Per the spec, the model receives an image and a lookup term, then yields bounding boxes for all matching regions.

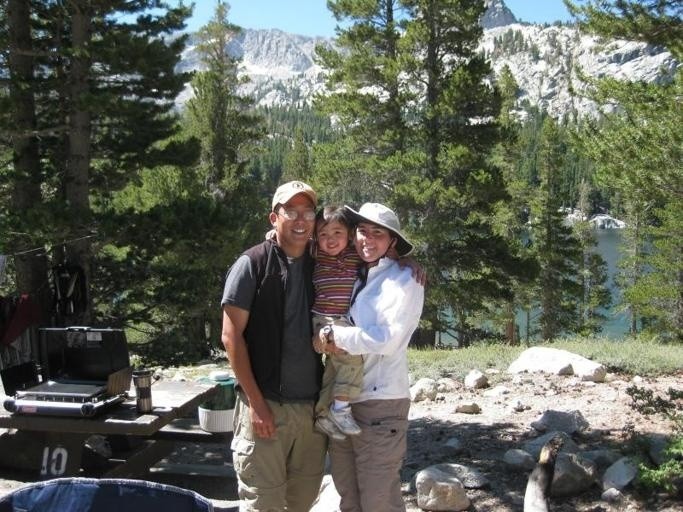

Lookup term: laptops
[13,326,128,403]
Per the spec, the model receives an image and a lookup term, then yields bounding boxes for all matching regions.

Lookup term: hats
[271,181,318,210]
[344,202,415,257]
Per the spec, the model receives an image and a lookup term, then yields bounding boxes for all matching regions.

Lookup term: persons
[219,179,428,512]
[263,203,399,443]
[312,202,424,512]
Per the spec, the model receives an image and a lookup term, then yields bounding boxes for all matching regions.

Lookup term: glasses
[274,210,317,221]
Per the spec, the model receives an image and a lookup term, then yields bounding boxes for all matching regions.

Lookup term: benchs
[0,418,232,446]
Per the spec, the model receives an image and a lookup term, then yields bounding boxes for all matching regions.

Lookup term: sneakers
[314,403,363,440]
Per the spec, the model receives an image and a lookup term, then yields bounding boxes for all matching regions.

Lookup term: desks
[0,380,221,480]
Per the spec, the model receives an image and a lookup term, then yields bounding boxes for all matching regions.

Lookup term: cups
[131,370,157,413]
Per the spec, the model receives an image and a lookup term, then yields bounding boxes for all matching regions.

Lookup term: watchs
[321,323,333,343]
